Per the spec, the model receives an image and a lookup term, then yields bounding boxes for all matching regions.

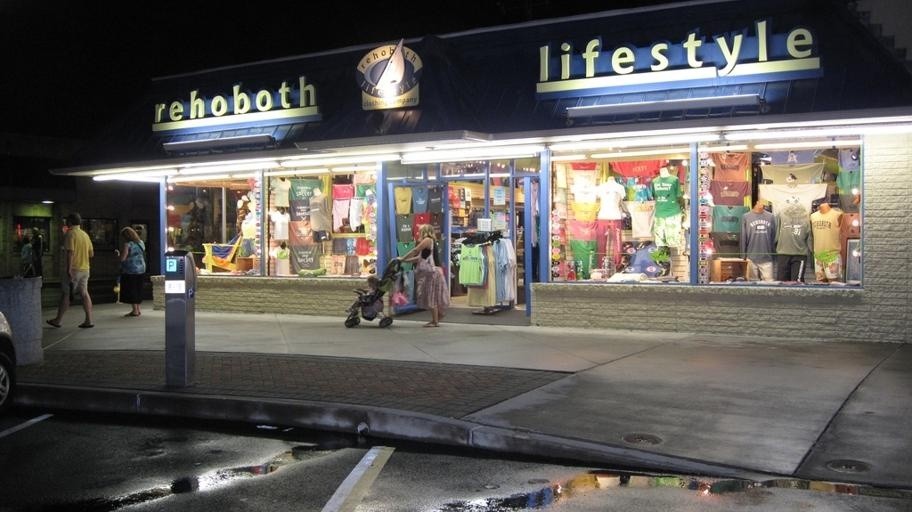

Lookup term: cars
[0,309,19,411]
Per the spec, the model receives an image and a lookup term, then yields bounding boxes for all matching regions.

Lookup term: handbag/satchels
[417,237,436,273]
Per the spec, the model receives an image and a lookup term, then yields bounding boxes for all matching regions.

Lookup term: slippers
[77,320,95,329]
[45,319,62,328]
[123,311,141,318]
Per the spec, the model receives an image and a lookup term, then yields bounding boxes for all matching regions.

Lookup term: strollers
[340,257,403,330]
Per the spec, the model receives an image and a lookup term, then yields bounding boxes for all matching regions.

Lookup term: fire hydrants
[112,277,124,304]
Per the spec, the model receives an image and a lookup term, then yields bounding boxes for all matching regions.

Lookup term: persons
[652,168,682,246]
[742,201,843,285]
[20,211,147,328]
[241,212,256,256]
[361,189,377,239]
[270,178,292,207]
[345,276,378,313]
[309,188,332,243]
[273,242,290,275]
[595,177,626,270]
[271,208,291,240]
[396,223,449,327]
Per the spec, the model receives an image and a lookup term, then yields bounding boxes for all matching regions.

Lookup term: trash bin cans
[0,274,44,367]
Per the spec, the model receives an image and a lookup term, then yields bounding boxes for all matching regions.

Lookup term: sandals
[425,321,439,328]
